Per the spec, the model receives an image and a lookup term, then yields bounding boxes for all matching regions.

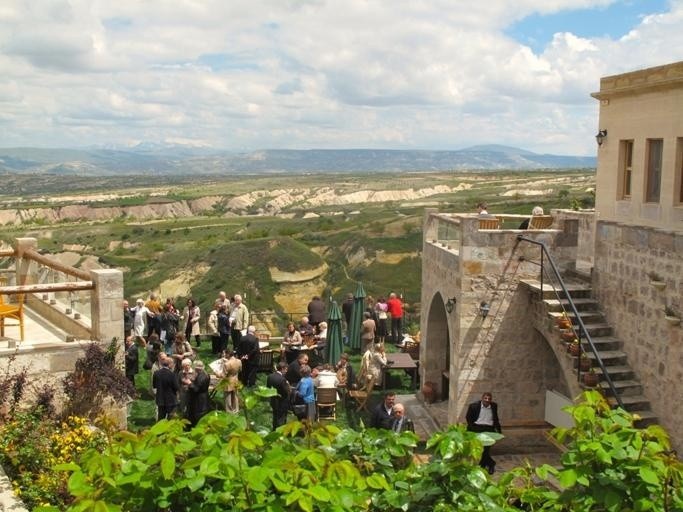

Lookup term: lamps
[441,293,458,317]
[595,129,608,146]
[478,301,489,318]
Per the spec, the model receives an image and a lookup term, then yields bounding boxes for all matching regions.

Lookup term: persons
[268,294,328,430]
[465,392,503,476]
[475,201,498,230]
[517,205,544,230]
[343,292,403,351]
[123,291,259,423]
[335,343,415,433]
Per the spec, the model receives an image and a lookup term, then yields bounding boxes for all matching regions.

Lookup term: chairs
[0,276,24,341]
[525,216,553,229]
[478,218,500,231]
[200,326,422,421]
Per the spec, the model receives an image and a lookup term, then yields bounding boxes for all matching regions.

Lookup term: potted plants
[558,312,615,411]
